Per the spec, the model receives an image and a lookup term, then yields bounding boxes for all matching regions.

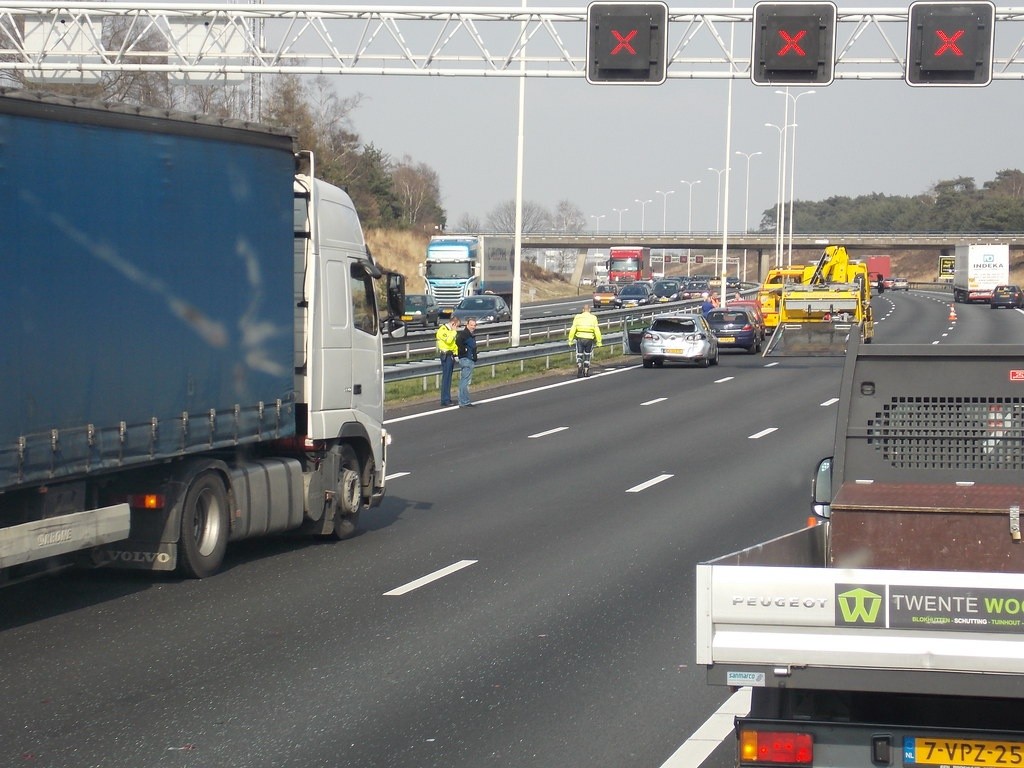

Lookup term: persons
[701,291,719,319]
[436,316,461,406]
[735,291,744,301]
[569,304,602,377]
[455,319,477,408]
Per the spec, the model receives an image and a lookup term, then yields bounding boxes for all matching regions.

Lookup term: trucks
[695,325,1024,768]
[418,235,514,315]
[0,71,405,578]
[950,244,1009,304]
[594,247,653,288]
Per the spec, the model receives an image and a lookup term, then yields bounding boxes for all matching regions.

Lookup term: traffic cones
[948,304,957,320]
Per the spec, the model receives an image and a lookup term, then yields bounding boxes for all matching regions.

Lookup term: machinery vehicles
[758,246,884,344]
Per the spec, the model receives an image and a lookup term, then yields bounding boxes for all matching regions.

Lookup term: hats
[702,292,709,302]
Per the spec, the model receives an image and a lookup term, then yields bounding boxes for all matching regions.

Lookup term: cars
[614,274,740,310]
[723,300,767,341]
[990,285,1024,309]
[402,294,440,326]
[706,307,762,355]
[592,284,619,308]
[883,278,909,291]
[622,313,719,368]
[449,295,510,335]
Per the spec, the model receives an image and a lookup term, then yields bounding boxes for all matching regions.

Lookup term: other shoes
[464,404,475,408]
[584,373,589,376]
[577,368,582,378]
[448,402,455,406]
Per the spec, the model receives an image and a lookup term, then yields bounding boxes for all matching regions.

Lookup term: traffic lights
[906,0,996,87]
[585,0,669,85]
[751,1,838,87]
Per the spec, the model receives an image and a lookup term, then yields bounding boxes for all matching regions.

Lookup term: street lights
[612,90,818,282]
